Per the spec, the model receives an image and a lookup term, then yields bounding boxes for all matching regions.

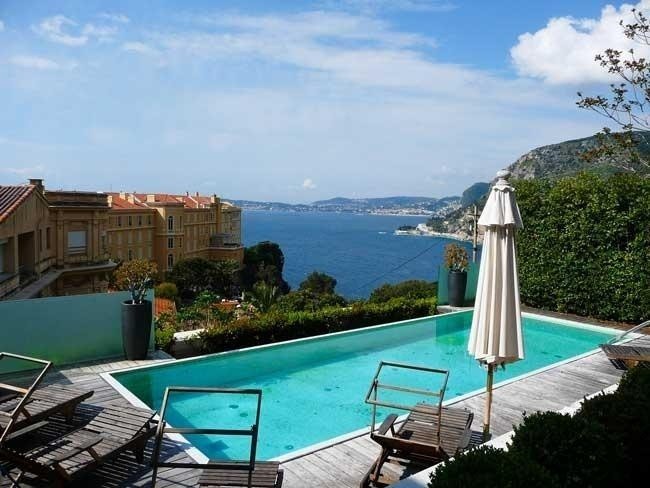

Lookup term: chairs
[148,386,284,488]
[599,320,650,370]
[0,403,167,487]
[359,361,475,488]
[0,351,95,475]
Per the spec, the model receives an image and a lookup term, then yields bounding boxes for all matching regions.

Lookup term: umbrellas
[467,171,525,444]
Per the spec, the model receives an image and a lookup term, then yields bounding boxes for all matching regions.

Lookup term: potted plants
[113,256,154,360]
[442,242,472,307]
